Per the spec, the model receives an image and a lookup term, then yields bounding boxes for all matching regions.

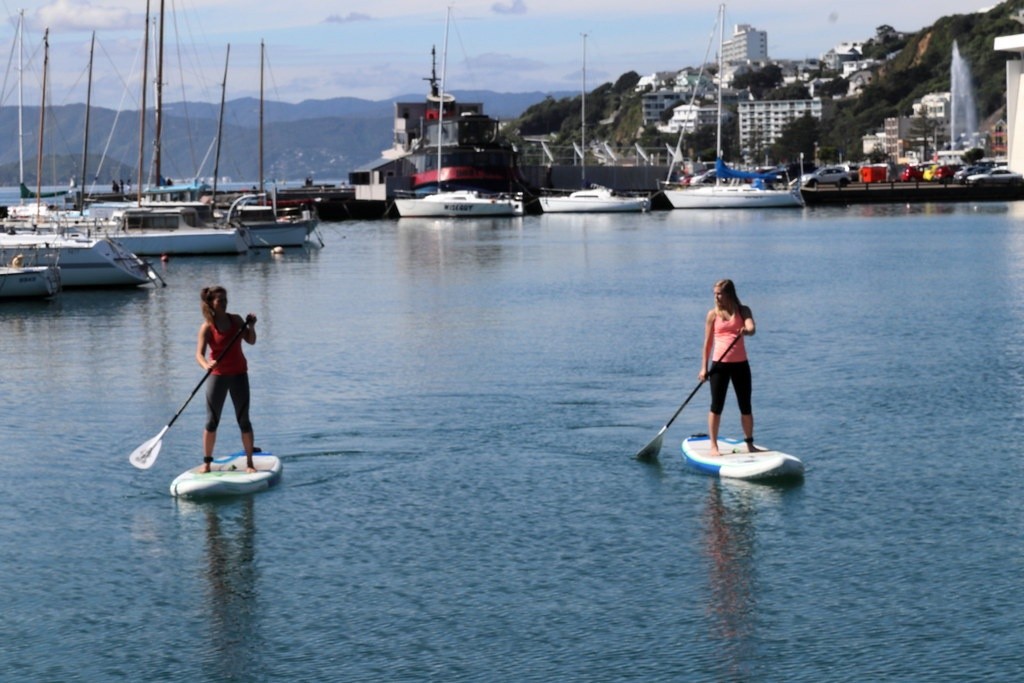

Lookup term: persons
[196,286,258,474]
[111,174,174,198]
[698,279,763,456]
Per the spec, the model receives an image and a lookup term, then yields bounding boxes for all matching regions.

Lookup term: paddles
[127,316,252,470]
[637,329,743,460]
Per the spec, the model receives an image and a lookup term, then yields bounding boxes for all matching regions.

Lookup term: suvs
[798,166,852,188]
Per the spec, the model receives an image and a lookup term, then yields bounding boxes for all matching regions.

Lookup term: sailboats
[538,30,652,213]
[661,3,804,207]
[396,5,515,220]
[1,1,316,297]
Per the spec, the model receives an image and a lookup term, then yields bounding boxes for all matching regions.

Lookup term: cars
[899,165,931,181]
[678,163,817,187]
[936,165,966,183]
[966,167,1022,186]
[955,165,992,184]
[923,165,946,182]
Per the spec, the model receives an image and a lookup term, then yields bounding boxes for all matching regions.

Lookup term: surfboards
[169,446,282,501]
[679,432,806,482]
